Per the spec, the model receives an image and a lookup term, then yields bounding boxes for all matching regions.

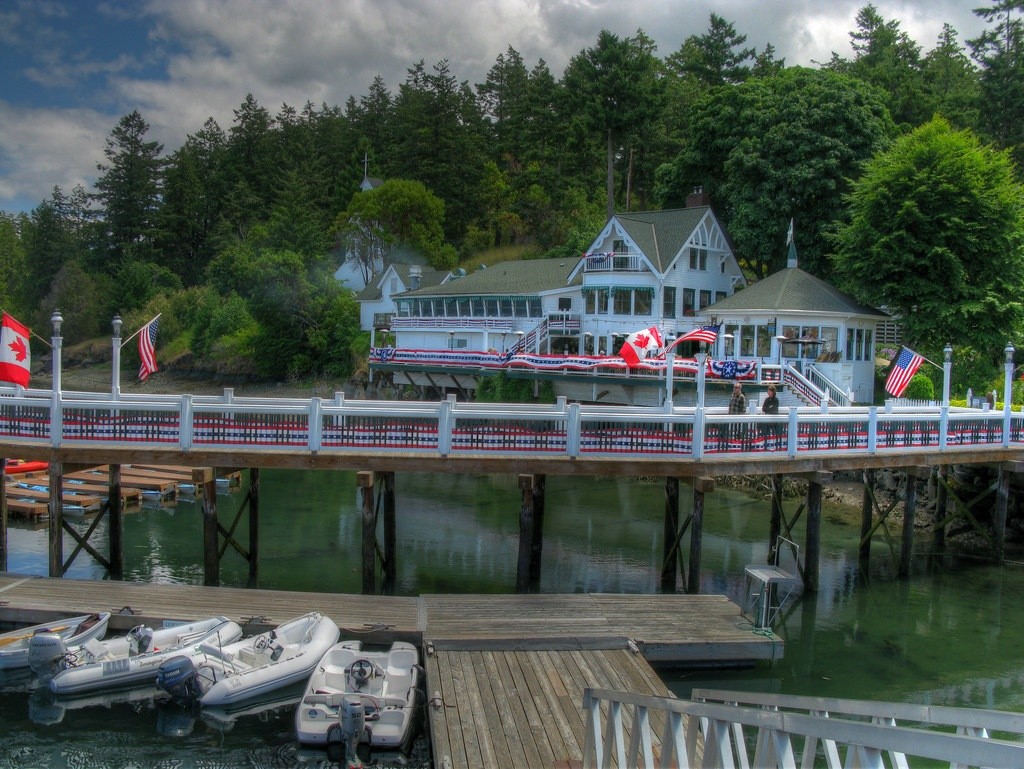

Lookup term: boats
[27,616,241,695]
[0,610,112,677]
[294,634,420,769]
[156,609,342,706]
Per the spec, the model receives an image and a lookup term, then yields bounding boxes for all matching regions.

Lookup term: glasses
[733,386,739,389]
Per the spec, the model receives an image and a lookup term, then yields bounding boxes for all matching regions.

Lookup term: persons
[762,385,779,415]
[987,392,994,410]
[728,382,746,415]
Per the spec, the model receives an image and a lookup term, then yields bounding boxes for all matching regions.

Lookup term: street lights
[513,331,524,353]
[611,333,618,356]
[744,335,752,352]
[721,334,734,361]
[694,325,708,458]
[500,330,509,354]
[380,329,390,350]
[581,331,592,354]
[939,342,955,450]
[620,332,630,341]
[448,330,456,350]
[1002,340,1015,449]
[772,335,789,365]
[666,328,678,414]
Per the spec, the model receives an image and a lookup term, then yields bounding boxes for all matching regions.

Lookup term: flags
[0,312,31,390]
[582,252,614,262]
[785,217,793,247]
[885,348,924,399]
[657,325,720,357]
[137,317,159,381]
[618,325,663,369]
